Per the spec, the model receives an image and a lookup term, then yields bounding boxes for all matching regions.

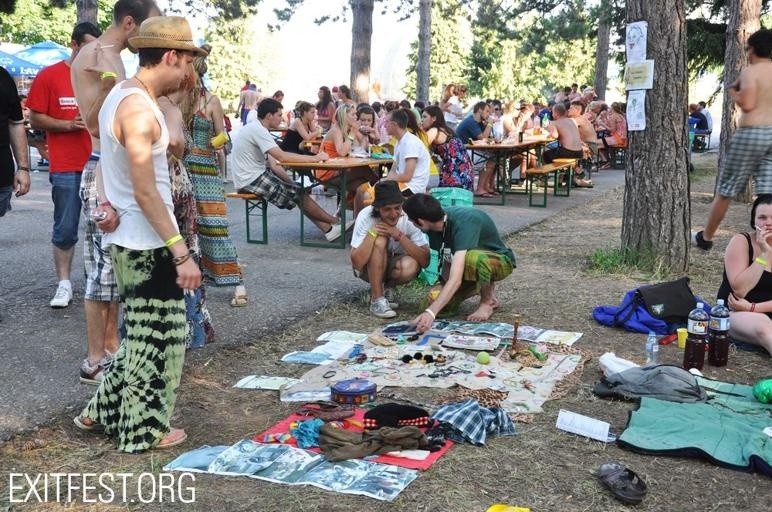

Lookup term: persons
[1,65,30,217]
[627,25,643,51]
[688,103,708,151]
[25,22,103,306]
[101,45,115,49]
[716,193,772,358]
[71,0,162,385]
[19,94,51,161]
[696,28,771,251]
[175,42,252,308]
[403,193,516,334]
[154,55,214,350]
[696,101,713,134]
[350,180,432,319]
[73,15,206,453]
[214,78,631,246]
[628,99,641,118]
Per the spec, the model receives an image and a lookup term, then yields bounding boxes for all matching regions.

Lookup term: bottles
[707,298,731,368]
[644,329,660,365]
[683,302,710,371]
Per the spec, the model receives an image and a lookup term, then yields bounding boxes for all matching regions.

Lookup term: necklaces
[428,365,472,378]
[133,75,161,111]
[372,366,401,382]
[164,95,176,107]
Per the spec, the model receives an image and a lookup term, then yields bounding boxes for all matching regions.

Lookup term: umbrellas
[0,40,43,77]
[11,38,74,68]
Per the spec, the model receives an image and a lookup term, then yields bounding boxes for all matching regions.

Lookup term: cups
[676,327,688,349]
[210,131,229,146]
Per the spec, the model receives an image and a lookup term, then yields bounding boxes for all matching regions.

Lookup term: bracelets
[100,201,110,206]
[16,165,30,172]
[172,253,191,265]
[755,257,768,267]
[323,370,337,379]
[164,234,183,247]
[100,72,117,79]
[750,302,755,312]
[425,308,436,320]
[503,378,517,387]
[728,85,736,90]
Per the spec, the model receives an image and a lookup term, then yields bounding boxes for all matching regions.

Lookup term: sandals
[598,463,647,505]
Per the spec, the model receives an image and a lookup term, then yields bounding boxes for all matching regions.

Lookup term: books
[441,333,501,352]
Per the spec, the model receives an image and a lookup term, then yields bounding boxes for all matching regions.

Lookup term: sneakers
[299,175,313,186]
[51,287,73,308]
[324,220,354,242]
[518,179,535,187]
[691,230,713,250]
[79,357,113,385]
[370,296,397,318]
[383,287,399,308]
[334,217,354,232]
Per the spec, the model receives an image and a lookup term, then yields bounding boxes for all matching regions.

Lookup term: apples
[476,351,490,364]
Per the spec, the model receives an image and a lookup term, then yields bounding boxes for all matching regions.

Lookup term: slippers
[156,428,187,449]
[474,193,493,197]
[73,416,94,430]
[230,293,249,307]
[488,191,499,195]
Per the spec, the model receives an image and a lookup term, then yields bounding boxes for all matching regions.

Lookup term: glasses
[343,103,351,112]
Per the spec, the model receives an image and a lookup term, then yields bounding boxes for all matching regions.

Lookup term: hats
[372,180,403,207]
[128,16,208,56]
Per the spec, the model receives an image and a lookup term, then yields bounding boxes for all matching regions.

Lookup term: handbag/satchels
[593,363,708,403]
[635,276,698,323]
